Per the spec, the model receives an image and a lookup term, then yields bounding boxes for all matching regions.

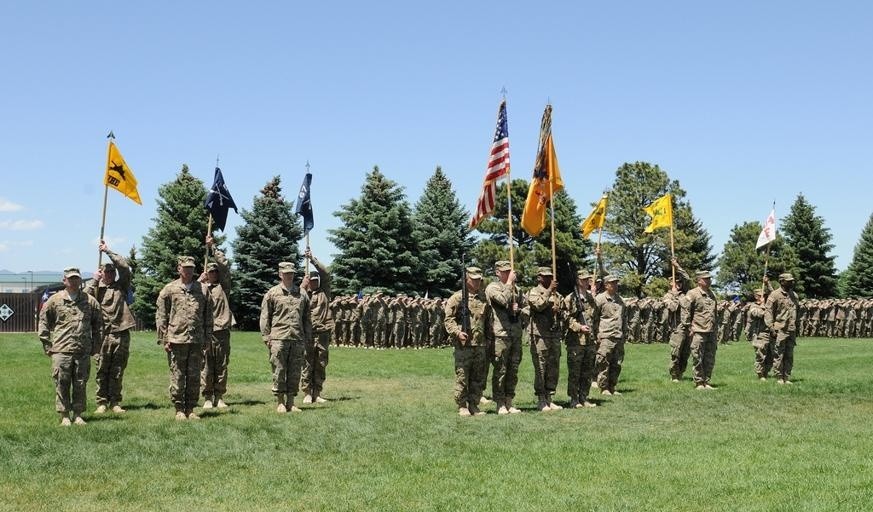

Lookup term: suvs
[37,283,65,314]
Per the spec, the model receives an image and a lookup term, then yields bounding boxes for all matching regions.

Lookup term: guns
[456,253,467,350]
[567,263,590,345]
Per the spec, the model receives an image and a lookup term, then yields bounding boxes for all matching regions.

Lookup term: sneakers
[329,343,454,351]
[759,372,768,382]
[175,408,200,421]
[58,411,87,427]
[214,393,228,407]
[776,375,793,385]
[695,381,712,390]
[95,402,126,416]
[203,395,213,408]
[303,393,327,404]
[278,399,305,415]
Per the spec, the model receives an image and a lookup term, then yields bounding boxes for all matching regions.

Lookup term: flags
[105,142,143,205]
[755,209,776,249]
[520,104,563,237]
[580,197,607,238]
[204,167,238,232]
[39,288,50,311]
[642,194,672,233]
[295,174,314,236]
[464,100,512,233]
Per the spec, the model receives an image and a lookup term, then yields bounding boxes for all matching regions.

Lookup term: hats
[207,262,219,273]
[577,270,593,280]
[753,288,766,297]
[64,266,82,280]
[278,262,296,273]
[603,274,620,285]
[332,290,447,308]
[466,267,483,281]
[798,297,872,305]
[495,260,511,272]
[309,271,319,281]
[536,267,553,276]
[668,275,682,284]
[777,273,795,282]
[625,296,662,306]
[178,255,196,268]
[101,262,115,272]
[716,300,741,307]
[695,270,713,281]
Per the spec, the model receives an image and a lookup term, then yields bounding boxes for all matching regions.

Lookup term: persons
[260,262,313,413]
[298,250,329,404]
[82,240,137,414]
[195,236,237,408]
[155,256,214,421]
[38,266,102,427]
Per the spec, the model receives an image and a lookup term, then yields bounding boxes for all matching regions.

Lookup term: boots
[537,394,563,411]
[570,395,595,408]
[496,398,521,414]
[672,373,684,383]
[600,385,622,396]
[458,402,486,417]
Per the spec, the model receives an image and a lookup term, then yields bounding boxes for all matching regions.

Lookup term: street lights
[22,277,26,293]
[27,270,33,291]
